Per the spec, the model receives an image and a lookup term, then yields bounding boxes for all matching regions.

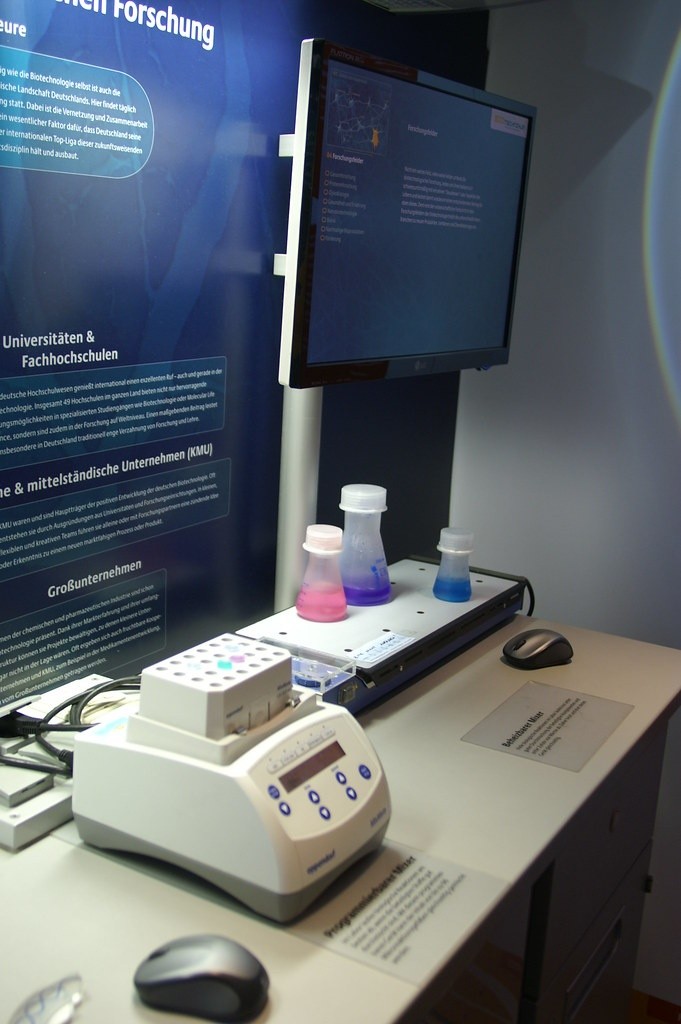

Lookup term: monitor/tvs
[277,37,536,387]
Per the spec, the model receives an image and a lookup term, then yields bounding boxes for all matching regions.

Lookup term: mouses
[134,933,270,1024]
[502,629,573,669]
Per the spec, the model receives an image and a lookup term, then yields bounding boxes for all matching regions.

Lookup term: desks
[0,616,681,1024]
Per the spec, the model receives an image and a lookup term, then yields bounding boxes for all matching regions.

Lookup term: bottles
[295,523,348,622]
[338,483,392,607]
[433,528,474,602]
